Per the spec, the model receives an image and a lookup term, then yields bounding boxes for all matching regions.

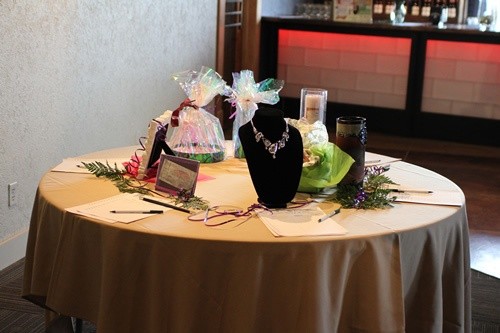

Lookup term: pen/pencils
[318,208,341,222]
[110,210,164,213]
[365,160,381,163]
[76,165,86,168]
[389,188,434,193]
[143,197,192,214]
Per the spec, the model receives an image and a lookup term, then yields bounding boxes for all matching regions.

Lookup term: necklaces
[251,118,290,159]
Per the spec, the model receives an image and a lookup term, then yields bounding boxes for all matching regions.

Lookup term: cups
[294,3,329,19]
[336,115,366,189]
[300,87,327,125]
[477,8,493,32]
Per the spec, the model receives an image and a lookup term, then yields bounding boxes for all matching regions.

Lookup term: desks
[20,140,472,333]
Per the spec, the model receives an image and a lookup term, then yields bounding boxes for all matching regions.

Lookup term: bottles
[373,1,456,24]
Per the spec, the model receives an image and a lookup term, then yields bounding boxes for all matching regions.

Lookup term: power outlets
[9,181,18,207]
